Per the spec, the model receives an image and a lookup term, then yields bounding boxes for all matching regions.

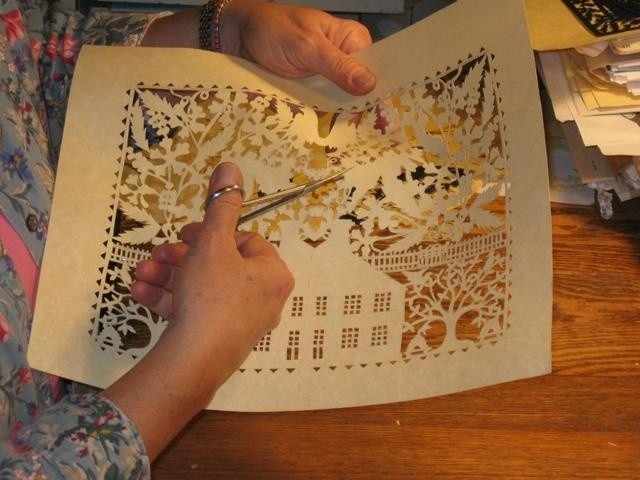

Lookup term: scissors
[204,166,354,231]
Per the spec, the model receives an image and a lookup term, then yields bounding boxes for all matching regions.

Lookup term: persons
[0,1,376,480]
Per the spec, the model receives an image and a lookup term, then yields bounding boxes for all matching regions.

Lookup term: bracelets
[197,0,228,54]
